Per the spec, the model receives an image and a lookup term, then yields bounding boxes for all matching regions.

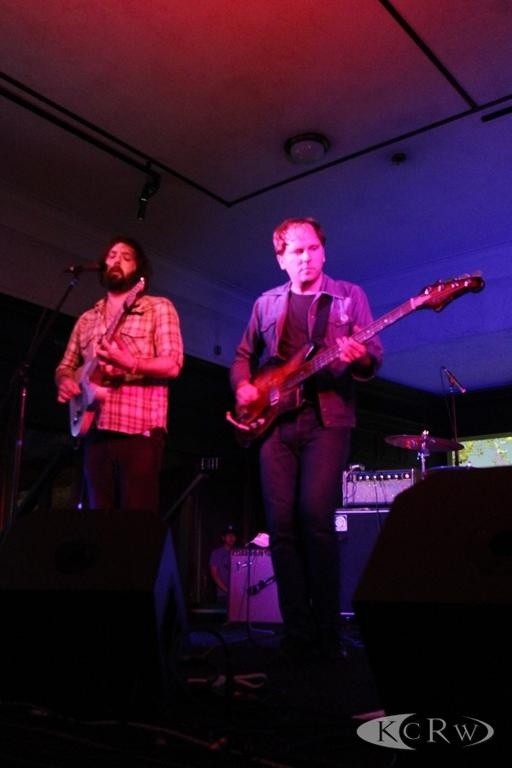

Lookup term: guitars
[227,269,486,444]
[69,276,145,436]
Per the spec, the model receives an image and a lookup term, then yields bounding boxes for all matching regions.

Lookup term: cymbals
[385,433,464,454]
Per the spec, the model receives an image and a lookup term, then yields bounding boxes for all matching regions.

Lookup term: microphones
[440,366,467,394]
[62,262,107,273]
[248,579,264,595]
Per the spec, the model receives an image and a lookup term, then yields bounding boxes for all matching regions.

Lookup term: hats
[246,533,271,548]
[224,525,238,533]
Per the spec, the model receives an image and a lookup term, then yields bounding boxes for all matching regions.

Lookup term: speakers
[351,469,511,732]
[1,506,189,717]
[228,547,284,631]
[334,507,391,616]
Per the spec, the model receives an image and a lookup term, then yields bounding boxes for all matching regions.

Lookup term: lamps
[285,132,330,165]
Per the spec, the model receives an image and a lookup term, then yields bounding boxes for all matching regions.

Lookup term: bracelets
[130,358,138,375]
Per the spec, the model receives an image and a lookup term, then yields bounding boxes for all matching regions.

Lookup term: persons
[209,522,239,607]
[229,218,381,717]
[53,236,185,511]
